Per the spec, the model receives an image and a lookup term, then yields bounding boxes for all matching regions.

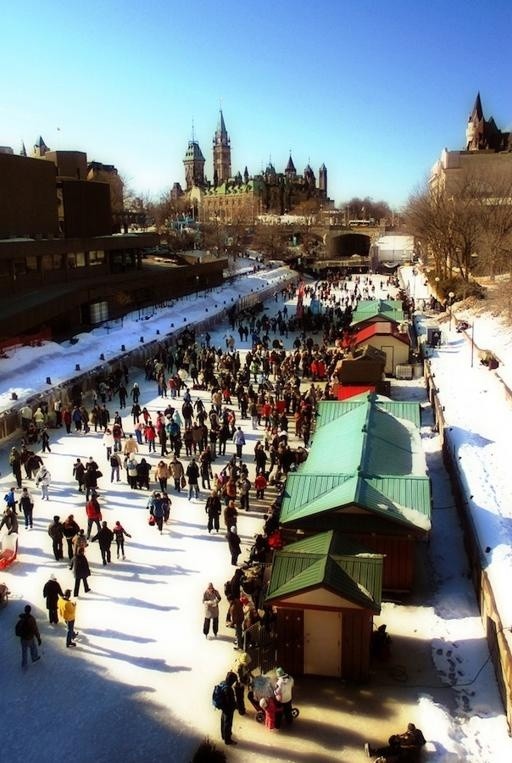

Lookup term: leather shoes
[226,740,237,744]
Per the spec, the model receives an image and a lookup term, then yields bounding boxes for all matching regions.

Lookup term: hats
[48,574,57,581]
[259,698,267,710]
[277,668,284,677]
[240,652,252,664]
[63,589,72,596]
[240,597,248,603]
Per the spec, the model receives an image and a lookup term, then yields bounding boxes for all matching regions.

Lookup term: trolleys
[0,531,18,570]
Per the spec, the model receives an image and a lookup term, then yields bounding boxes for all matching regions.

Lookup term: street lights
[435,277,440,299]
[448,292,455,331]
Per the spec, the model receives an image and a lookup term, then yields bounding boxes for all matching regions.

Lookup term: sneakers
[67,643,76,648]
[366,742,373,757]
[73,632,79,640]
[32,656,40,664]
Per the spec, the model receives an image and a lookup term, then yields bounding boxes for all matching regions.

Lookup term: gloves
[38,638,41,645]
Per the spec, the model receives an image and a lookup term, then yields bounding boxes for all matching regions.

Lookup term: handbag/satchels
[148,514,155,526]
[38,472,47,482]
[181,476,186,488]
[275,689,282,702]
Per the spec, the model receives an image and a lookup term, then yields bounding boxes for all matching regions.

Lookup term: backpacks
[15,614,33,637]
[113,426,121,437]
[213,684,231,709]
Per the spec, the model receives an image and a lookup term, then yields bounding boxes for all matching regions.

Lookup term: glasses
[208,587,213,590]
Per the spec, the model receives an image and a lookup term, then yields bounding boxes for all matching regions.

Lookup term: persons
[69,549,91,596]
[427,290,464,311]
[72,458,86,491]
[156,404,182,459]
[10,444,22,489]
[146,490,172,535]
[69,529,88,570]
[135,423,144,444]
[44,575,65,624]
[289,302,319,338]
[255,474,267,499]
[29,455,44,477]
[34,397,49,429]
[155,460,169,493]
[145,421,156,452]
[187,459,199,501]
[112,521,132,560]
[230,304,291,337]
[373,624,392,646]
[481,355,499,369]
[244,532,268,565]
[36,465,52,501]
[109,448,122,482]
[55,399,62,428]
[0,507,18,535]
[82,466,102,501]
[91,521,114,565]
[137,458,152,490]
[316,270,414,341]
[40,429,51,453]
[130,401,142,424]
[253,426,308,473]
[219,423,228,456]
[124,453,130,483]
[201,583,222,639]
[169,456,184,492]
[26,423,38,442]
[237,474,251,511]
[116,384,129,409]
[456,322,468,334]
[213,672,238,744]
[182,388,208,456]
[260,698,284,731]
[225,500,238,533]
[205,491,223,533]
[22,447,32,479]
[199,446,214,490]
[220,407,235,426]
[123,434,139,453]
[64,515,79,558]
[224,569,246,627]
[274,284,315,303]
[210,405,219,429]
[19,487,35,530]
[5,487,18,512]
[102,429,114,460]
[101,405,110,433]
[79,407,90,434]
[223,387,232,405]
[143,426,149,443]
[86,457,99,471]
[57,590,78,647]
[225,595,244,650]
[17,605,42,666]
[263,484,285,533]
[144,359,181,399]
[232,427,246,458]
[140,407,151,424]
[213,463,238,500]
[237,334,348,430]
[210,426,218,461]
[225,526,241,565]
[127,452,139,489]
[116,365,129,382]
[274,668,294,731]
[20,405,32,431]
[231,653,252,715]
[73,406,82,432]
[114,411,124,436]
[366,724,426,763]
[92,374,116,404]
[48,516,65,561]
[113,422,123,451]
[63,407,72,434]
[91,405,101,431]
[230,454,248,474]
[86,494,102,539]
[166,326,249,390]
[129,382,140,403]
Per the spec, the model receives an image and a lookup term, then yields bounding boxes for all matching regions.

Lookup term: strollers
[247,680,300,722]
